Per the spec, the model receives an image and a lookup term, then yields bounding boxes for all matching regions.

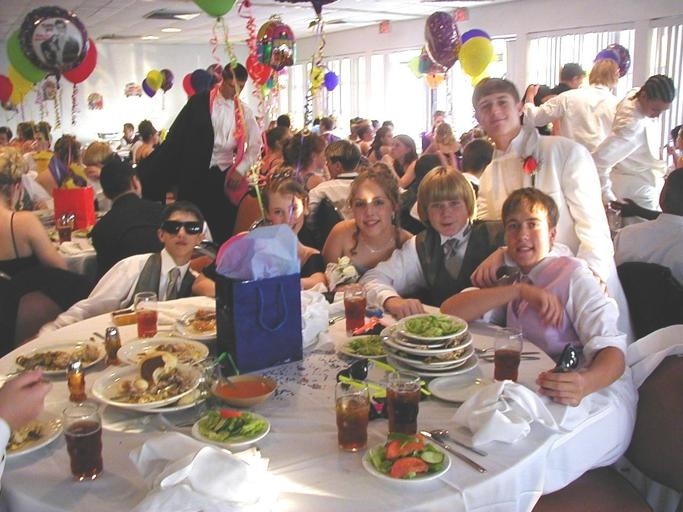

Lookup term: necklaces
[361,236,395,255]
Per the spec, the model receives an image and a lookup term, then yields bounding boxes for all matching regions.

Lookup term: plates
[337,335,387,359]
[210,375,277,407]
[176,308,216,340]
[12,342,106,373]
[5,414,62,457]
[91,364,207,412]
[191,412,270,447]
[116,337,209,366]
[380,314,478,377]
[428,376,493,402]
[362,440,451,482]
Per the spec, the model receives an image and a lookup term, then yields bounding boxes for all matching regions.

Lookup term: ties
[512,277,532,315]
[164,269,181,302]
[445,241,463,277]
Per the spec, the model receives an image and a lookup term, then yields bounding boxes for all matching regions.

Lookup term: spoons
[430,429,487,456]
[473,347,493,353]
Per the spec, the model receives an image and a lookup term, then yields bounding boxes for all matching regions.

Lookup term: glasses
[163,220,201,235]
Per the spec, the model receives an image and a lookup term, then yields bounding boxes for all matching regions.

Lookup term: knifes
[419,430,486,473]
[479,351,539,358]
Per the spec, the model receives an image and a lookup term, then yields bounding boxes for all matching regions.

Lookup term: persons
[440,187,640,470]
[522,59,620,156]
[309,140,364,215]
[319,163,416,285]
[378,135,422,235]
[0,145,71,344]
[51,136,81,183]
[424,124,463,158]
[522,63,586,132]
[422,111,446,150]
[0,367,53,481]
[593,74,675,219]
[474,78,633,346]
[37,199,217,336]
[367,129,396,162]
[264,126,295,179]
[82,142,114,196]
[120,123,139,143]
[135,121,160,160]
[317,118,334,141]
[276,115,290,132]
[0,121,52,158]
[351,117,394,139]
[665,127,682,169]
[282,133,326,187]
[359,167,504,322]
[248,165,324,292]
[460,137,495,191]
[91,160,171,276]
[188,64,263,244]
[614,168,683,287]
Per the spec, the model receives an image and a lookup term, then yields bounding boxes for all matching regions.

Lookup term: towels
[300,291,329,348]
[458,381,557,446]
[132,433,268,501]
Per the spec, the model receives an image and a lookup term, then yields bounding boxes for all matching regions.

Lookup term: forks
[485,356,539,362]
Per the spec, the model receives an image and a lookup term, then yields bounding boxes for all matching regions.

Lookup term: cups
[493,328,523,383]
[57,219,74,245]
[388,375,418,434]
[343,288,367,331]
[62,405,103,480]
[335,381,368,451]
[134,292,158,338]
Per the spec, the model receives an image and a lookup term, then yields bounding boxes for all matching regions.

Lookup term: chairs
[0,267,90,357]
[318,199,345,250]
[617,261,683,341]
[531,324,683,512]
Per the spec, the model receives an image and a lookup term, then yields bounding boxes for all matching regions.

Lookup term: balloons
[323,71,338,92]
[424,11,460,73]
[160,69,173,93]
[19,6,90,91]
[260,77,278,96]
[457,29,493,84]
[310,68,325,88]
[246,50,272,86]
[146,70,162,92]
[141,78,156,98]
[256,22,270,56]
[263,23,282,64]
[272,25,295,71]
[190,70,211,95]
[193,0,236,22]
[310,85,319,96]
[0,75,12,107]
[7,66,34,108]
[6,28,49,86]
[62,38,97,87]
[207,64,222,83]
[182,73,195,96]
